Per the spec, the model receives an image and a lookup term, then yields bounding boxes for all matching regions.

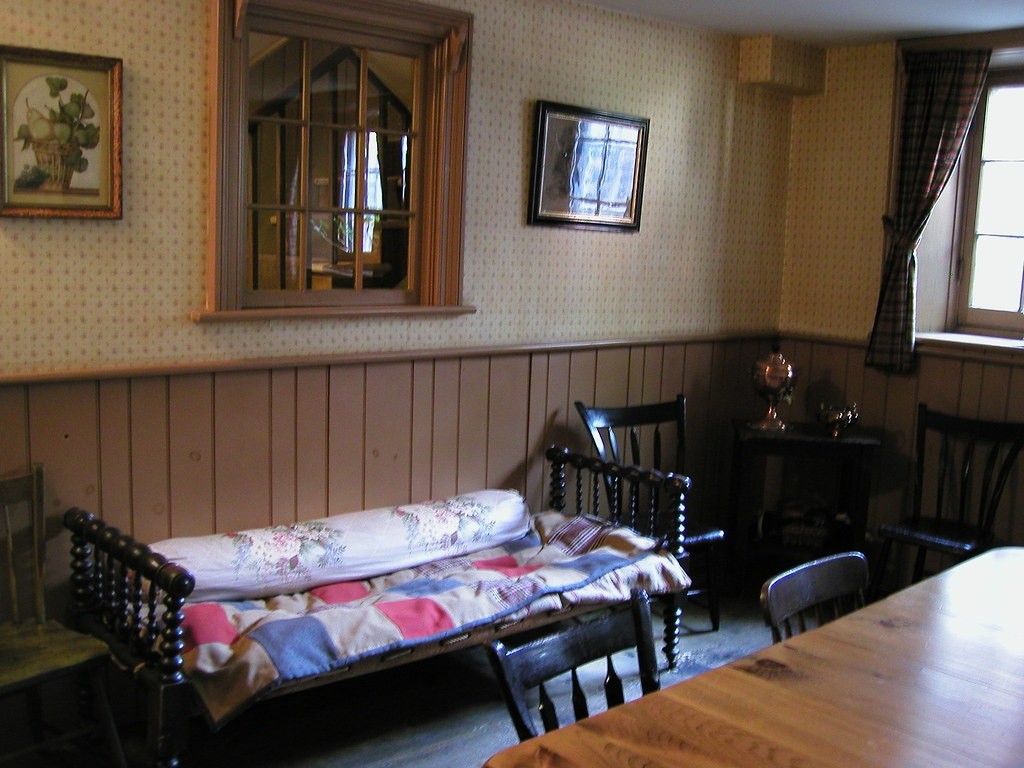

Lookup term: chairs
[867,401,1024,605]
[759,551,870,646]
[484,585,660,742]
[576,392,728,632]
[0,461,129,768]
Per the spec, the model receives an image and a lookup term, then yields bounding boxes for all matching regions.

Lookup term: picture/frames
[0,43,124,221]
[526,98,651,233]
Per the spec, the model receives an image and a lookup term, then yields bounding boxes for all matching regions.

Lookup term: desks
[491,544,1024,768]
[720,417,883,608]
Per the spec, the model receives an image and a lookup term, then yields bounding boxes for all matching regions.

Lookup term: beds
[60,444,694,768]
